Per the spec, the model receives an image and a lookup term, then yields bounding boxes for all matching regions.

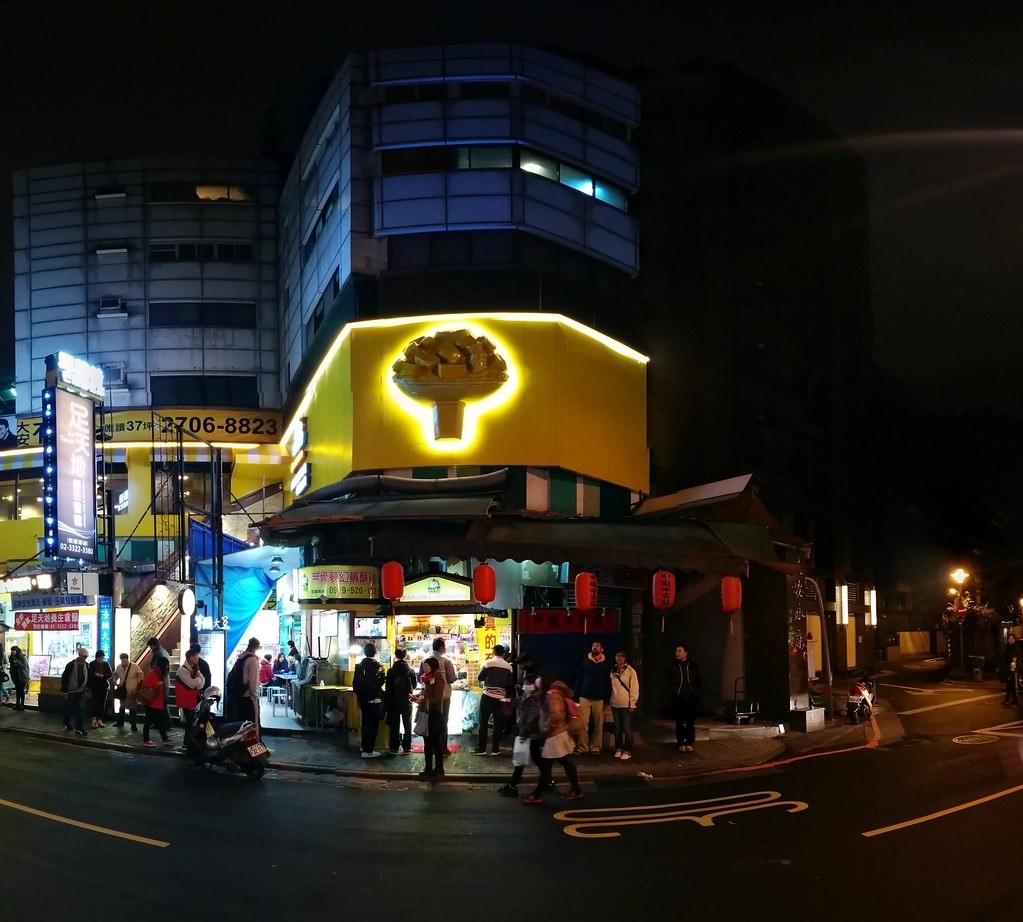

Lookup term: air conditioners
[103,368,124,385]
[100,297,121,310]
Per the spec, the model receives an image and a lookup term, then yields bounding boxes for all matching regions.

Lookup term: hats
[78,649,89,656]
[501,643,510,652]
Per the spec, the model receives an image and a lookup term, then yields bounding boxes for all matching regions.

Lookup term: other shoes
[521,794,541,803]
[360,747,502,757]
[13,704,25,710]
[182,745,187,750]
[678,745,693,752]
[1002,699,1017,705]
[576,751,601,755]
[419,766,445,778]
[614,752,631,759]
[143,737,174,746]
[560,790,584,800]
[64,718,138,734]
[5,698,11,704]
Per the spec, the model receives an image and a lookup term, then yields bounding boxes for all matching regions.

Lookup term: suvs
[997,639,1023,690]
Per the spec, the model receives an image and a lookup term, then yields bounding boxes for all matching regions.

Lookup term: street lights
[950,568,969,667]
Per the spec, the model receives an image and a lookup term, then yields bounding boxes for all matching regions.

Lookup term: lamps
[270,555,284,564]
[270,566,281,573]
[311,535,319,563]
[320,594,328,605]
[368,536,375,560]
[290,593,299,603]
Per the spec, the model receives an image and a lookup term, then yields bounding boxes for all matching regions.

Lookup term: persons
[1001,633,1023,704]
[497,674,560,797]
[10,637,640,760]
[666,646,699,752]
[520,671,584,804]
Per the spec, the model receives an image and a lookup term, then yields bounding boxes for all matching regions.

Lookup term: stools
[260,685,289,717]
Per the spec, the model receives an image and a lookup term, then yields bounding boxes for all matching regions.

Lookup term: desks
[276,674,298,707]
[311,686,354,728]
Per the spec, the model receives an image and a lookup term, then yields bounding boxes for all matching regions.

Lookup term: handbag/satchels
[414,698,444,739]
[635,698,642,713]
[511,735,532,767]
[113,684,159,706]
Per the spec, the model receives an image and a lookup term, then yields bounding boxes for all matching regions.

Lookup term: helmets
[203,686,222,701]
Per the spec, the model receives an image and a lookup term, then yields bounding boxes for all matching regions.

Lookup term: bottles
[320,680,324,688]
[407,633,430,641]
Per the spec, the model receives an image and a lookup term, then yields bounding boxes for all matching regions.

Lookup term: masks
[520,665,541,693]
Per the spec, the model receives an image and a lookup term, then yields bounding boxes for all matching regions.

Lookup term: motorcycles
[846,669,878,724]
[178,699,271,781]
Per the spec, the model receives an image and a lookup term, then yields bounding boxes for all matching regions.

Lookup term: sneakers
[547,780,555,788]
[498,784,519,793]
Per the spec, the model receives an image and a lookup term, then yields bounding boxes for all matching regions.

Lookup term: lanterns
[652,570,676,632]
[722,575,742,635]
[473,562,496,605]
[574,571,598,636]
[382,560,403,603]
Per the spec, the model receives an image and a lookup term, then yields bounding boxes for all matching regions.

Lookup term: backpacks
[226,654,254,700]
[355,664,413,702]
[567,710,584,735]
[0,664,9,683]
